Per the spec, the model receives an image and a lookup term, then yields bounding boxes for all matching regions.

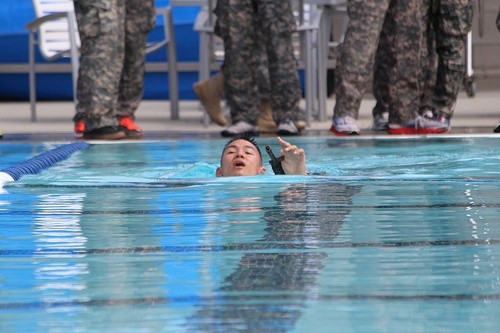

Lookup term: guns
[267,145,285,176]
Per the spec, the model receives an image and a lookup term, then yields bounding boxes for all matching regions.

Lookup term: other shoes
[84,125,143,140]
[436,110,450,131]
[118,115,138,130]
[75,116,86,133]
[369,111,390,130]
[418,105,437,120]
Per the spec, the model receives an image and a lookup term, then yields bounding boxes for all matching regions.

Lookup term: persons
[215,134,308,176]
[71,0,156,139]
[192,0,306,137]
[330,0,475,137]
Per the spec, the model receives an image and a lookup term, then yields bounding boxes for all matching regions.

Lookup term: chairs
[26,0,178,121]
[192,0,330,122]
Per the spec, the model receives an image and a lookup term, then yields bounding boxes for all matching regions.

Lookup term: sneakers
[387,109,448,134]
[276,117,300,134]
[331,114,360,135]
[221,120,260,137]
